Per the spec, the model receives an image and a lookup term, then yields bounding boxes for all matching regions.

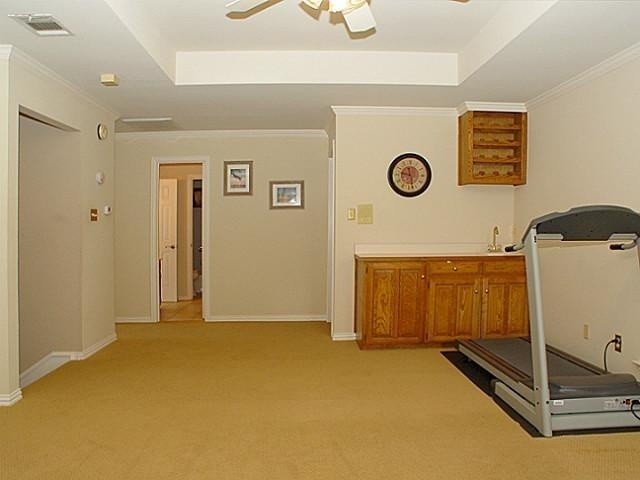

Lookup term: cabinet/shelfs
[354,257,426,350]
[458,111,527,186]
[424,257,482,348]
[481,256,530,340]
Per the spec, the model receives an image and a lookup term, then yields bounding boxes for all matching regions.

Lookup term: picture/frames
[269,180,305,209]
[224,160,253,196]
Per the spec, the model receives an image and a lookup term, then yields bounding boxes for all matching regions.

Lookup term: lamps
[301,0,365,13]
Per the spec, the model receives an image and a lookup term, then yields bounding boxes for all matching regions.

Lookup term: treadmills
[455,205,639,437]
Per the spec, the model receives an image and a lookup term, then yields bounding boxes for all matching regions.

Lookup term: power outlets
[614,334,621,353]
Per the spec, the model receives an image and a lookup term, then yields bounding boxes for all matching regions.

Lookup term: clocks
[386,152,433,199]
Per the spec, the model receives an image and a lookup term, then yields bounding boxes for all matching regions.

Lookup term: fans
[225,0,470,34]
